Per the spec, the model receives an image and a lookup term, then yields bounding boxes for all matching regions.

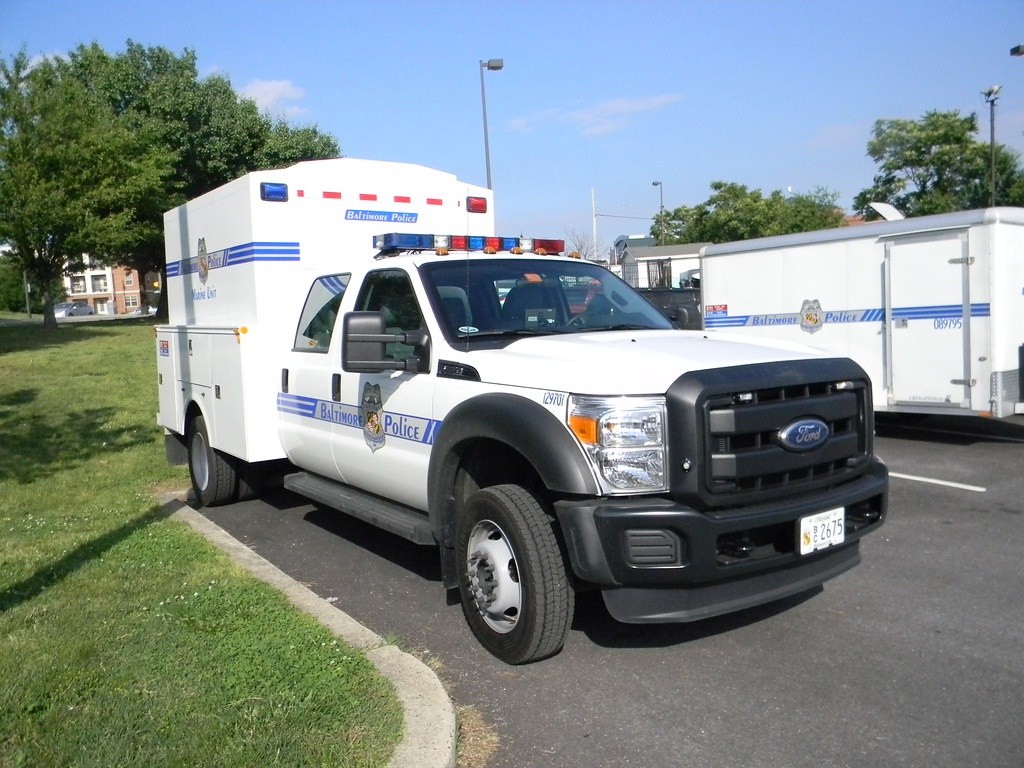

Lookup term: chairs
[305,294,468,361]
[500,287,525,312]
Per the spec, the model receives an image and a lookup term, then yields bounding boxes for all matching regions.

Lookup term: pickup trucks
[154,153,889,668]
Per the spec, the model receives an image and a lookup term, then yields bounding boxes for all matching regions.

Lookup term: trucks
[702,205,1024,440]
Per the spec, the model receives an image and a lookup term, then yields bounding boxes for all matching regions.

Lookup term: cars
[609,288,702,331]
[561,278,602,317]
[52,300,95,317]
[493,277,517,308]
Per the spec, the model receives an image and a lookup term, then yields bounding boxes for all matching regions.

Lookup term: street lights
[652,179,666,246]
[477,54,505,191]
[979,81,1001,206]
